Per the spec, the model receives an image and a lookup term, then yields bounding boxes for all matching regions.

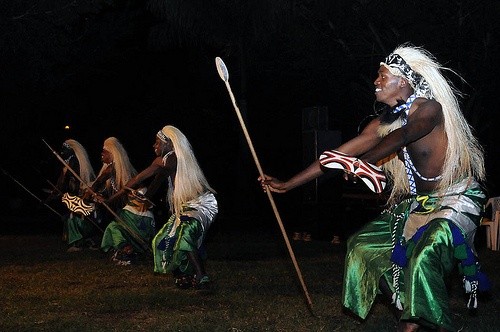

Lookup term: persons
[257,46,487,332]
[96,125,218,289]
[79,137,154,267]
[42,139,96,253]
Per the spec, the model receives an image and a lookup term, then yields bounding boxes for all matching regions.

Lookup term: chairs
[481,197,500,251]
